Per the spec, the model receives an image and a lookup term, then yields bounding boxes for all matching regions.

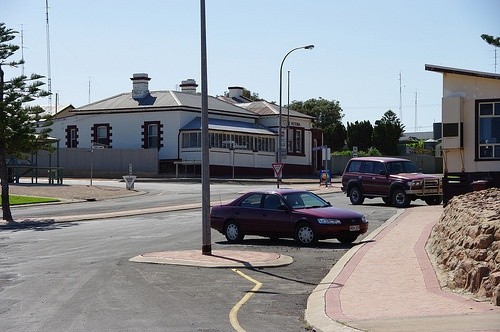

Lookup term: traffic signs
[92,146,104,150]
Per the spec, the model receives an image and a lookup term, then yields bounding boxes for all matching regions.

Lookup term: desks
[7,165,63,184]
[174,161,201,178]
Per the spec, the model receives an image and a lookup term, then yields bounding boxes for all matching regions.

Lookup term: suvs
[341,157,442,208]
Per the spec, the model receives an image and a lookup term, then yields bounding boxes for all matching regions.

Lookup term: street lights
[276,44,314,188]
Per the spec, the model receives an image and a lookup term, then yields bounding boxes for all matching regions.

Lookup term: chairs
[287,195,297,206]
[393,164,401,171]
[267,195,281,209]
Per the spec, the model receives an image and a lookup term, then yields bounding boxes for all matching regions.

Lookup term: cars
[210,189,368,247]
